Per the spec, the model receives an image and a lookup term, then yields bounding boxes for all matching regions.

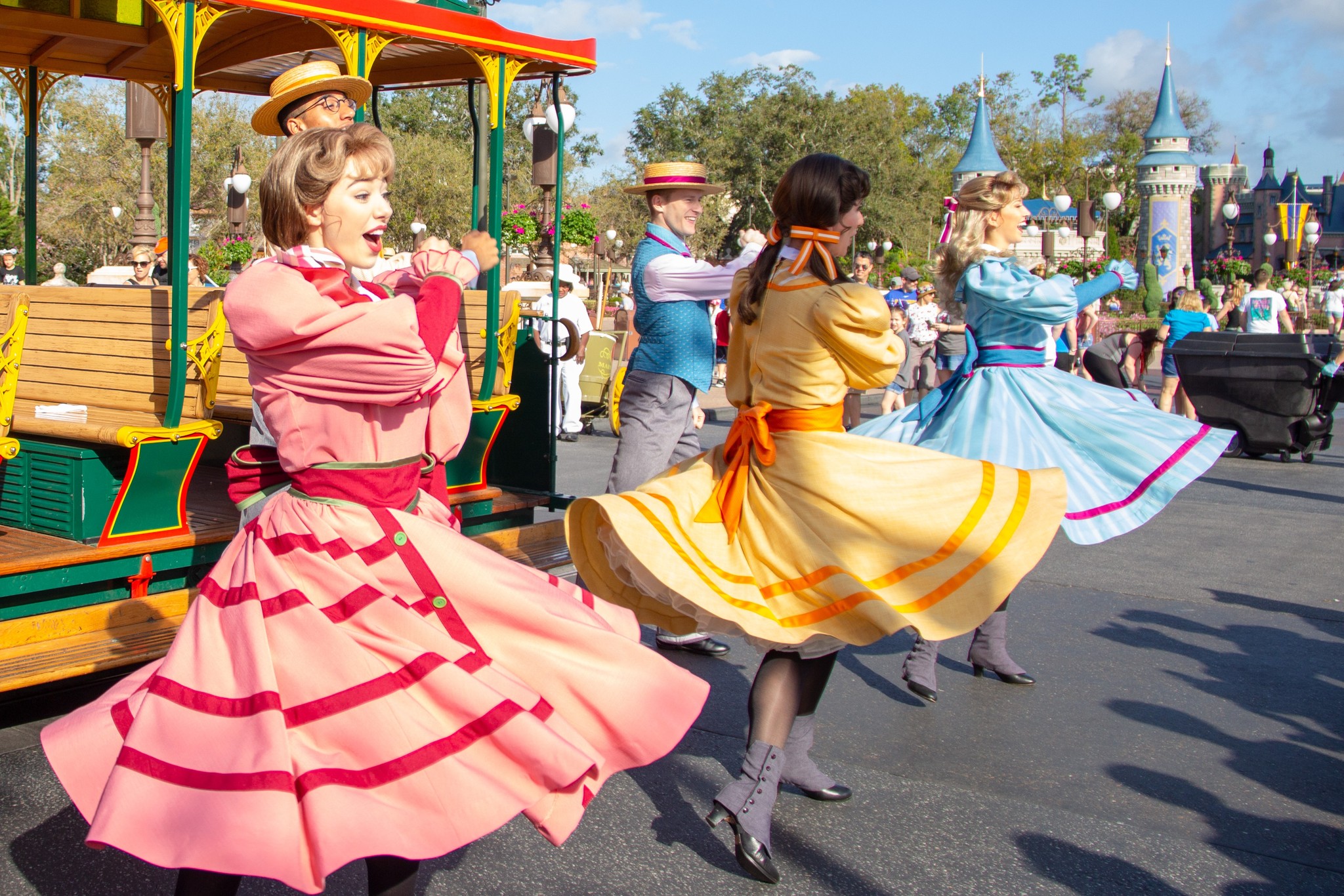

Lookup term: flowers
[1208,255,1253,278]
[500,201,600,246]
[1056,256,1108,279]
[219,234,254,272]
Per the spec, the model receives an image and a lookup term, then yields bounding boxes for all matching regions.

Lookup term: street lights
[410,204,429,251]
[1025,208,1070,283]
[1221,186,1241,258]
[597,224,624,302]
[868,235,894,286]
[224,142,252,242]
[1263,216,1307,270]
[1054,165,1125,281]
[521,69,575,282]
[1300,207,1322,307]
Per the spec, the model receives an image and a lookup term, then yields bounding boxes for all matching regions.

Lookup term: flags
[1276,203,1313,271]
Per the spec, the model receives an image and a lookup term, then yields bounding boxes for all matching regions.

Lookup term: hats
[547,262,581,284]
[623,161,725,196]
[900,266,923,280]
[890,276,903,286]
[250,60,373,137]
[154,236,168,254]
[915,281,938,298]
[617,288,628,295]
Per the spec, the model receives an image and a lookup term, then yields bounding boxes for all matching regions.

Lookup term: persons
[188,254,215,287]
[38,123,712,896]
[0,248,25,285]
[562,152,1070,883]
[239,60,499,530]
[847,171,1238,704]
[523,253,1344,458]
[123,244,160,285]
[152,237,168,285]
[573,162,769,655]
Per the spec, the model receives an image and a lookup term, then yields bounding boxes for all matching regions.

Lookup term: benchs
[212,293,522,420]
[0,284,226,447]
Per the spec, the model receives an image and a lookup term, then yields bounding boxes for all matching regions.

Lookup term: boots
[705,710,852,885]
[899,634,940,703]
[968,610,1037,685]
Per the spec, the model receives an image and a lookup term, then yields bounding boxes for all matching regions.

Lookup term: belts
[545,341,566,346]
[910,338,933,346]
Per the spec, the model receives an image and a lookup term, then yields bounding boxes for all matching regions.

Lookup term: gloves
[1322,359,1340,377]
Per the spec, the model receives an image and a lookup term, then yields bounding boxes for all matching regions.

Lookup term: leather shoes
[656,635,730,656]
[564,432,581,441]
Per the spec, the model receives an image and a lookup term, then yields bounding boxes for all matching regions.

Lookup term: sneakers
[715,379,727,387]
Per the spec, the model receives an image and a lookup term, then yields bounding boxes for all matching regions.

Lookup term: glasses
[188,266,199,273]
[130,260,151,268]
[1172,296,1178,301]
[292,96,358,119]
[1226,287,1233,290]
[853,263,870,271]
